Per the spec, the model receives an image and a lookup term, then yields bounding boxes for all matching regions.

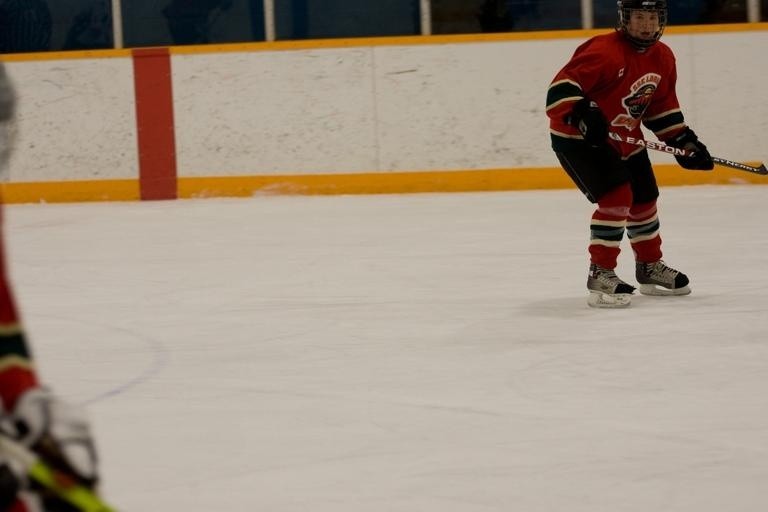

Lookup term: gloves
[562,98,611,146]
[664,126,714,170]
[0,387,96,499]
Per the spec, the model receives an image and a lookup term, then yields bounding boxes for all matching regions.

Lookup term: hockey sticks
[605,128,768,176]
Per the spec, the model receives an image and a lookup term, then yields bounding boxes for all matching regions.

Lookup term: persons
[1,67,101,511]
[543,2,715,296]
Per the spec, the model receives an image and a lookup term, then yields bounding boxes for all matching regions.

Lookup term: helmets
[613,0,669,46]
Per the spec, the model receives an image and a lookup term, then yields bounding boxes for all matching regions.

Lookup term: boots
[635,263,690,288]
[586,258,634,293]
